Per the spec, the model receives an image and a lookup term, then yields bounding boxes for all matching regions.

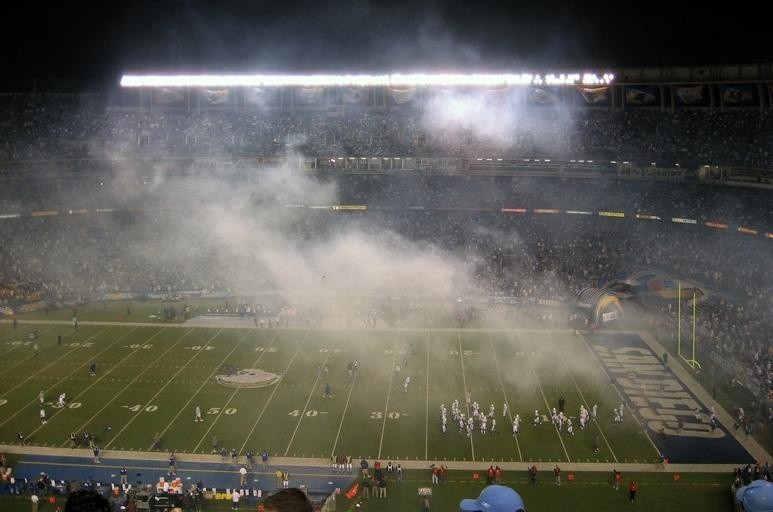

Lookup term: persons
[1,91,773,511]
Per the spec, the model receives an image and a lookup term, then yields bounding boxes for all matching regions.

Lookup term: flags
[147,79,653,108]
